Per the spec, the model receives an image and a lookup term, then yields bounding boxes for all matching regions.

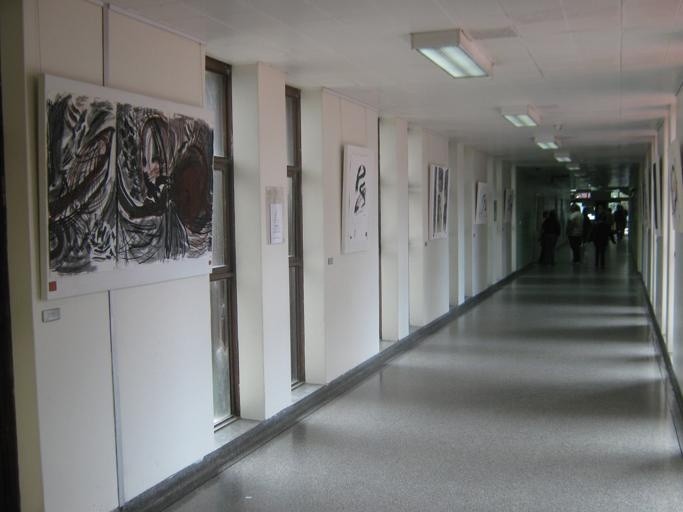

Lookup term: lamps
[410,28,581,173]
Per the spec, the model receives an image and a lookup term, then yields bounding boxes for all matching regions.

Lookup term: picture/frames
[475,181,493,228]
[635,139,683,242]
[502,187,515,222]
[340,142,377,254]
[428,164,451,241]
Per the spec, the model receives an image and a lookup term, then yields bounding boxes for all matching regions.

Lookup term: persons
[529,202,631,270]
